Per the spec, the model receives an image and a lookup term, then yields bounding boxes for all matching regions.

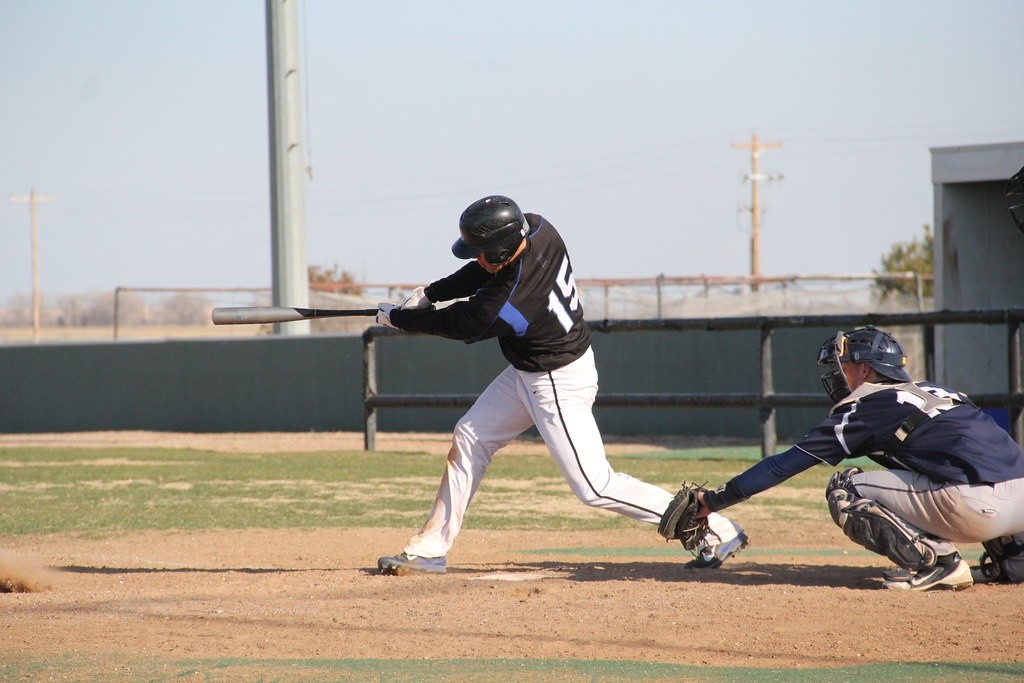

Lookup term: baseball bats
[212,304,436,327]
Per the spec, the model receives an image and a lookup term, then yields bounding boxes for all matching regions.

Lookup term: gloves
[401,287,432,310]
[376,303,401,331]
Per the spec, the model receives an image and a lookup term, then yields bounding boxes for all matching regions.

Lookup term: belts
[982,479,994,489]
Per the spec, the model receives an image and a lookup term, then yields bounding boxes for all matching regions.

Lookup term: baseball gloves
[654,486,710,551]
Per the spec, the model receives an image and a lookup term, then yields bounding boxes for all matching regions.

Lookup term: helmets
[452,195,530,265]
[838,324,913,382]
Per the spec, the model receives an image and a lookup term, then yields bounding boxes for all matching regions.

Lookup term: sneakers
[971,560,1005,583]
[882,551,973,590]
[378,552,446,575]
[684,532,750,569]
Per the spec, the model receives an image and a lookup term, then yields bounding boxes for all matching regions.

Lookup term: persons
[376,196,747,574]
[693,326,1024,592]
